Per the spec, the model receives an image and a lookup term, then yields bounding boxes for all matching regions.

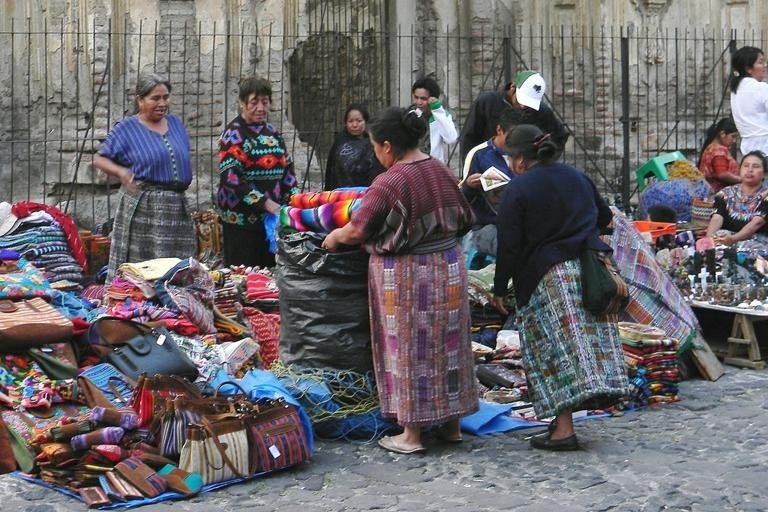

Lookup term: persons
[93,75,197,275]
[706,150,768,246]
[458,107,531,223]
[699,118,741,193]
[460,71,567,159]
[217,78,297,269]
[320,106,472,454]
[410,78,459,166]
[325,106,386,191]
[729,47,768,169]
[492,124,614,451]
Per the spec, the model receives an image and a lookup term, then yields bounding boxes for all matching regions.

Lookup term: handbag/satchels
[0,316,312,509]
[580,249,631,316]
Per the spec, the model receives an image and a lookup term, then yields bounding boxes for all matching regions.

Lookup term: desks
[684,284,768,370]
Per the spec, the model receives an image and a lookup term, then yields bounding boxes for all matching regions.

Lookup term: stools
[636,150,687,192]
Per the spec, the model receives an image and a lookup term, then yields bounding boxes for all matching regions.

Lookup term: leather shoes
[530,418,579,452]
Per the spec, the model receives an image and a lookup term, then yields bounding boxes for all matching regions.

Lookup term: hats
[513,70,546,112]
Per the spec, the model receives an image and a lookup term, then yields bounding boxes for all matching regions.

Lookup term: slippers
[378,435,427,454]
